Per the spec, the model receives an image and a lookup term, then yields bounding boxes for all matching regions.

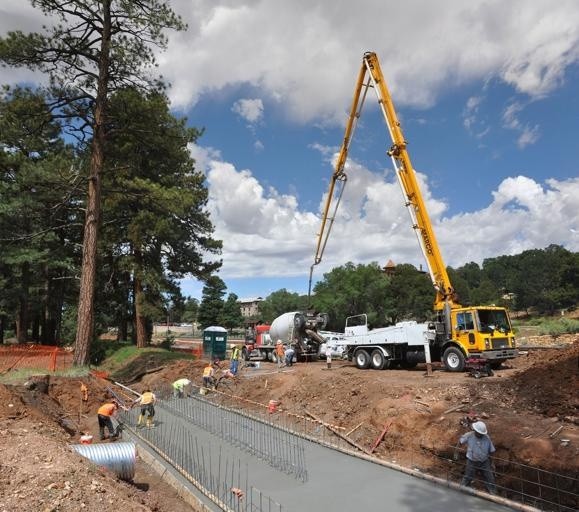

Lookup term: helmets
[471,420,489,435]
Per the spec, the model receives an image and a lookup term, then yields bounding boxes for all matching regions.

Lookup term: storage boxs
[80,435,93,444]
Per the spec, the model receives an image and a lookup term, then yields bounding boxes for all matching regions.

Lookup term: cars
[316,330,349,361]
[156,323,193,327]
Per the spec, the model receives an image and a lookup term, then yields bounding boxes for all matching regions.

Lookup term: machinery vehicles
[239,310,320,365]
[315,47,521,373]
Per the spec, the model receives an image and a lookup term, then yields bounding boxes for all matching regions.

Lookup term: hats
[230,343,236,349]
[276,340,282,345]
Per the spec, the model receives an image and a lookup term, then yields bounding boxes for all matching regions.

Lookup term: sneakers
[98,434,117,441]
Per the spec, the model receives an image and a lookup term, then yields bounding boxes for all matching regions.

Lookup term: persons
[173,378,192,398]
[131,390,157,427]
[325,346,332,368]
[203,364,214,388]
[276,339,285,364]
[458,421,496,495]
[97,400,119,440]
[283,349,295,366]
[230,344,240,375]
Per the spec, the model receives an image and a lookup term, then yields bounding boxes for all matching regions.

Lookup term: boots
[146,416,154,427]
[139,415,145,425]
